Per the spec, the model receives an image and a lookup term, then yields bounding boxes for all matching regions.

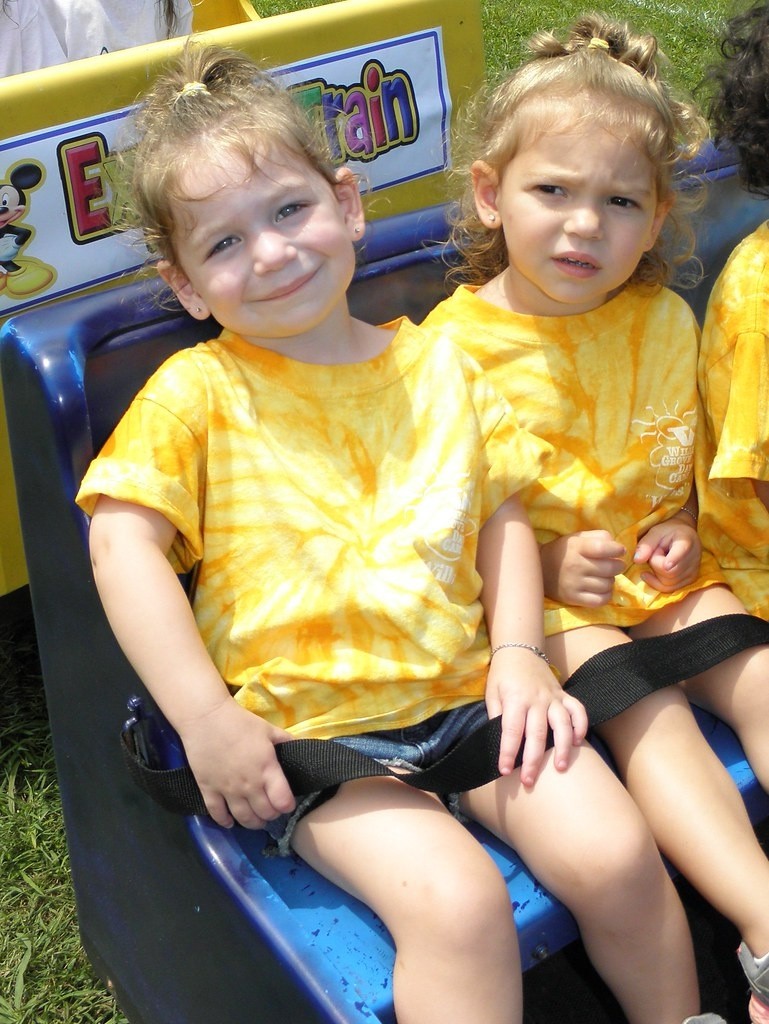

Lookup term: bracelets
[681,507,697,522]
[490,643,551,665]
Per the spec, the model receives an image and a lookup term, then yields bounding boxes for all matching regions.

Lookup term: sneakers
[736,936,769,1023]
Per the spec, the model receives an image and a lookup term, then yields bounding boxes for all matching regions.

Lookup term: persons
[75,53,732,1024]
[691,2,768,615]
[0,0,194,81]
[414,17,768,1021]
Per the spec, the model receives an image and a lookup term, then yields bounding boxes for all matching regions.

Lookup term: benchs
[0,133,769,1023]
